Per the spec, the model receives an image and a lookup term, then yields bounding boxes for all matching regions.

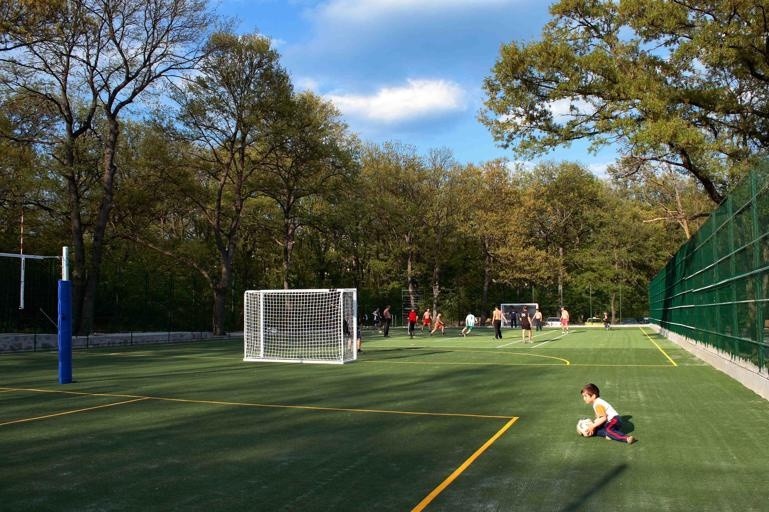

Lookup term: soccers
[576,418,595,437]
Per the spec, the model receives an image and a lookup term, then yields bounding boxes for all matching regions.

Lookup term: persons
[603,312,611,328]
[581,383,635,444]
[343,305,569,352]
